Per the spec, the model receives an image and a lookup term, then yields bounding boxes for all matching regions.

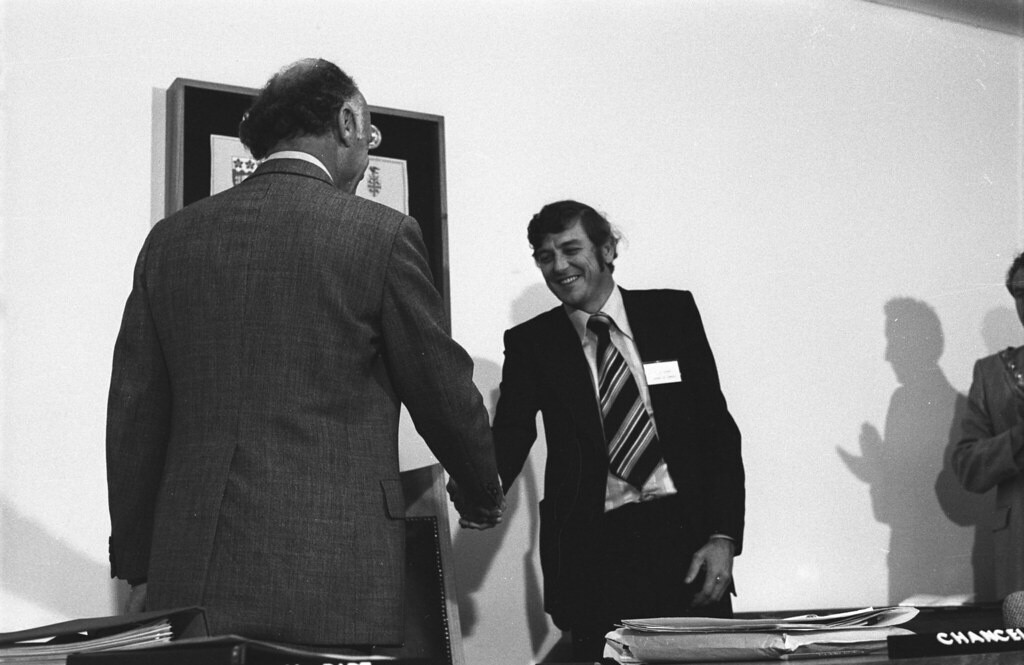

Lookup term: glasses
[352,123,382,150]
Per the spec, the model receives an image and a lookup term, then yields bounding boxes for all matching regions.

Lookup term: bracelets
[127,578,148,584]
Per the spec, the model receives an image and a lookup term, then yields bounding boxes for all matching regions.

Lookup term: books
[603,603,921,665]
[0,605,400,665]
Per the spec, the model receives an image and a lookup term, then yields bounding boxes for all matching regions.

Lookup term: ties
[586,314,663,492]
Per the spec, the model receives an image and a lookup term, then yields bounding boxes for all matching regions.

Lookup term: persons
[950,252,1024,604]
[445,199,745,665]
[106,58,503,655]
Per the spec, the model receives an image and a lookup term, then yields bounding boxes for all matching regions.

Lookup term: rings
[716,576,721,582]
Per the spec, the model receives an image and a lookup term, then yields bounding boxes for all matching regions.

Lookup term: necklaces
[1002,346,1024,388]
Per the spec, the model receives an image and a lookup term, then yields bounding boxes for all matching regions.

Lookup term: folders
[0,605,210,665]
